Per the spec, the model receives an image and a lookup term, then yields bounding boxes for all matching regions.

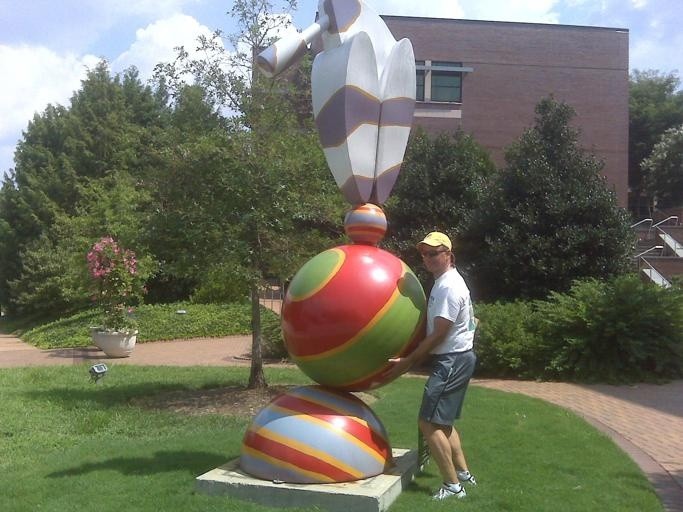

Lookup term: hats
[415,232,452,252]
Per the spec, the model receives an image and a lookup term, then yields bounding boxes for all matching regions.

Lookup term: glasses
[421,249,446,258]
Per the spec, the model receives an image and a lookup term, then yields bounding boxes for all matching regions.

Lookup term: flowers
[85,234,154,330]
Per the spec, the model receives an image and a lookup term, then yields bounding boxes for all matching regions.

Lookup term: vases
[87,327,142,361]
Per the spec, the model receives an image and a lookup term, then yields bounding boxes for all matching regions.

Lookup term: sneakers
[433,483,467,499]
[457,475,477,486]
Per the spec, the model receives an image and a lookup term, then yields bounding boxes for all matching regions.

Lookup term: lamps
[87,364,106,381]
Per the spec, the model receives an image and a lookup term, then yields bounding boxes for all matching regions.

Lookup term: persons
[379,230,477,501]
